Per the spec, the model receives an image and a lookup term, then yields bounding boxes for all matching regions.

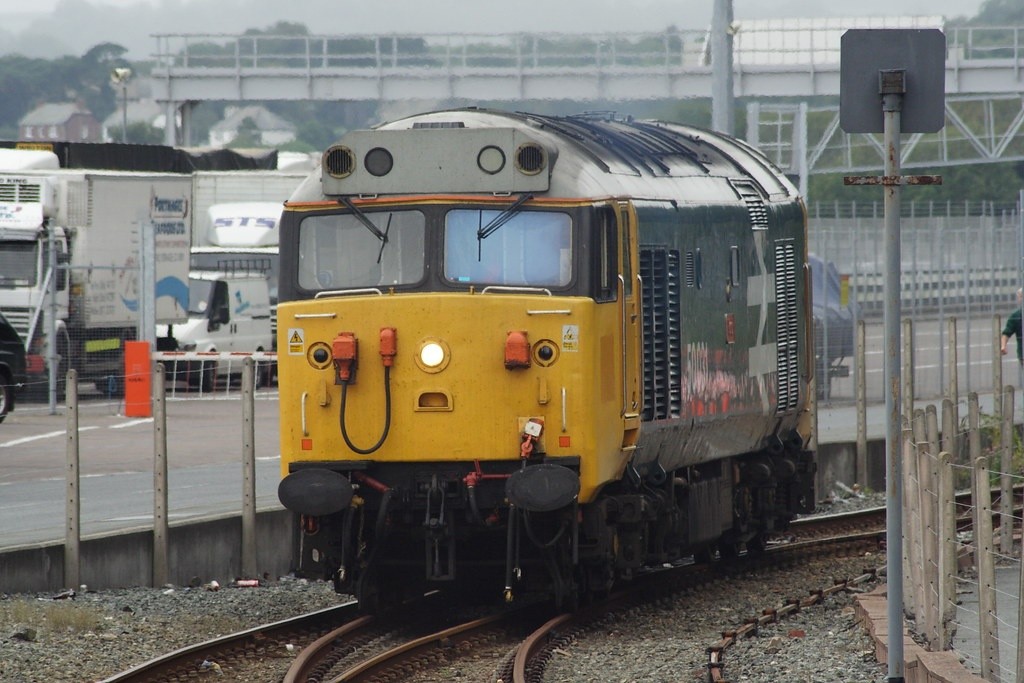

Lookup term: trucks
[156,150,325,391]
[0,139,195,423]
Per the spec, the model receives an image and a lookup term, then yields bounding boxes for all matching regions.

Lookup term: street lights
[111,67,131,144]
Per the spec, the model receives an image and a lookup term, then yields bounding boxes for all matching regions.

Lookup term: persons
[1000,288,1024,366]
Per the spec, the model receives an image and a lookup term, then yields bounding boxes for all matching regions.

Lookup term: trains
[276,97,861,619]
